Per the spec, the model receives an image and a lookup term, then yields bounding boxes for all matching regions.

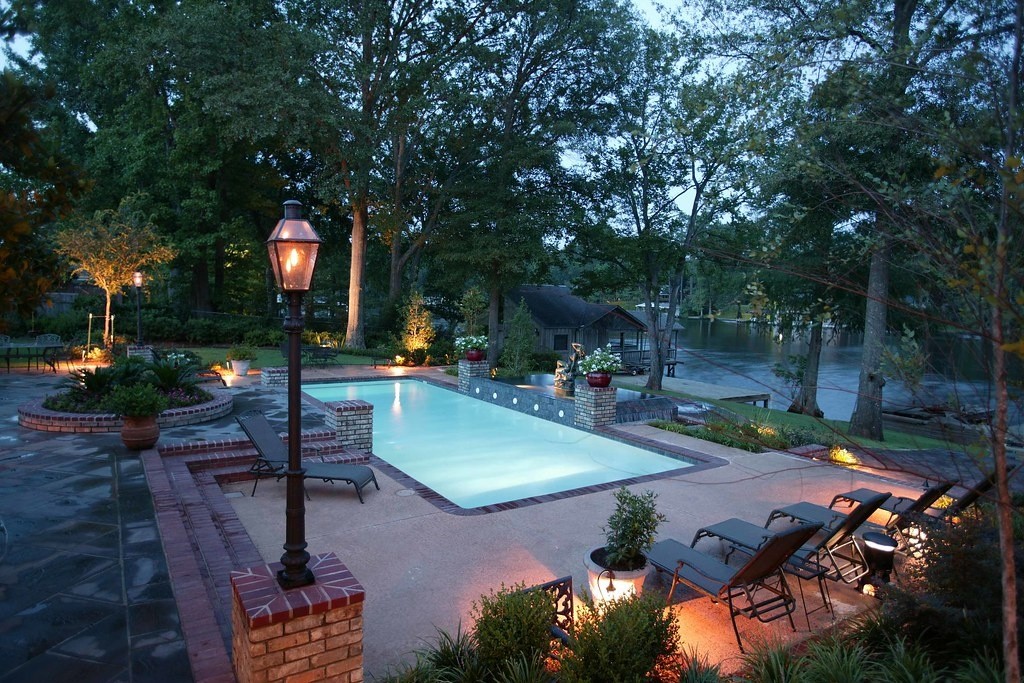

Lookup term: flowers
[455,335,489,353]
[577,343,622,375]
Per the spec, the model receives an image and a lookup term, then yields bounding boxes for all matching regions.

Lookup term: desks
[0,341,64,374]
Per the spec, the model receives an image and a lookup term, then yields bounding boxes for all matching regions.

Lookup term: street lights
[133,267,145,346]
[265,199,326,588]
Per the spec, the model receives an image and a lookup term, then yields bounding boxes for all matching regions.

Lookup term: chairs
[236,408,381,504]
[764,475,962,598]
[35,333,72,372]
[827,462,1017,561]
[690,492,894,632]
[637,521,825,654]
[0,334,11,374]
[280,341,343,369]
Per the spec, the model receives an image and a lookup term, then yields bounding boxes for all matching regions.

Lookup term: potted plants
[227,341,256,376]
[583,485,669,616]
[98,383,170,452]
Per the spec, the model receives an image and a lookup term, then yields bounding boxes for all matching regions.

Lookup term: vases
[585,372,612,387]
[465,350,483,361]
